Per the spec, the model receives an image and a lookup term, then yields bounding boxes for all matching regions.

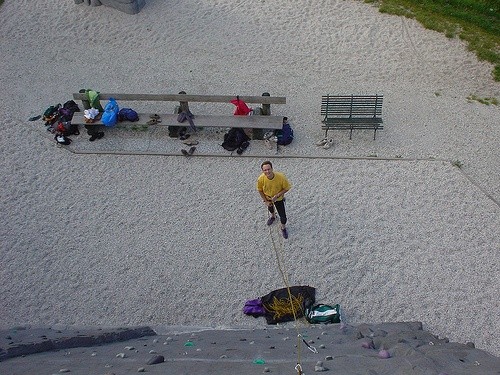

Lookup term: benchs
[71,92,286,139]
[321,95,386,141]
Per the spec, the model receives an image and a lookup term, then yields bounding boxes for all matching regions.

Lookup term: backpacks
[275,120,295,147]
[252,106,274,140]
[118,107,139,122]
[55,132,72,145]
[42,100,81,136]
[169,105,187,138]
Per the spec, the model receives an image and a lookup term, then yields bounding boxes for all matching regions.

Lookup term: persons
[255,160,293,240]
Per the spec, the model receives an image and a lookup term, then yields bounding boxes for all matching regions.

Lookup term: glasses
[180,134,190,140]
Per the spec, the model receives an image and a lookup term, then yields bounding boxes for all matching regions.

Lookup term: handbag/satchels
[305,302,341,323]
[223,125,249,151]
[100,97,119,128]
[258,285,316,325]
[231,99,253,115]
[242,297,265,318]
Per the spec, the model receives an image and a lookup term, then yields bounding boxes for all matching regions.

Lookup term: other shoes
[267,215,277,225]
[89,131,104,141]
[281,226,288,239]
[236,142,250,154]
[29,115,41,121]
[147,119,161,125]
[324,138,334,149]
[316,138,328,145]
[150,113,159,119]
[181,147,195,160]
